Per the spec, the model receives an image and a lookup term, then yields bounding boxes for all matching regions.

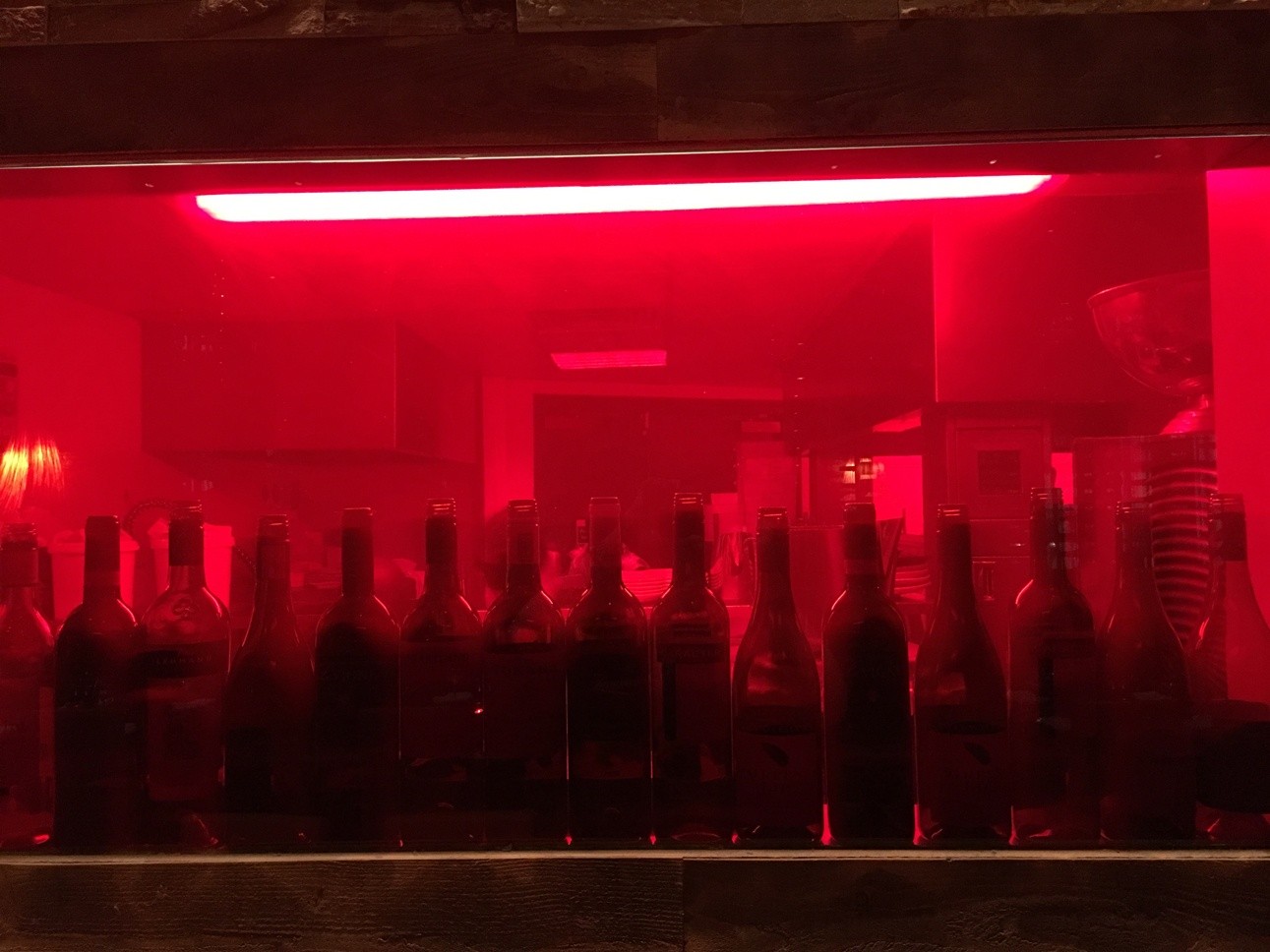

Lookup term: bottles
[732,507,824,850]
[1008,487,1095,849]
[311,507,399,852]
[483,496,566,851]
[52,512,138,855]
[648,489,732,849]
[227,512,315,853]
[914,502,1007,849]
[822,503,914,851]
[397,497,483,851]
[1184,492,1270,849]
[0,519,54,855]
[1094,498,1187,846]
[565,496,648,848]
[137,496,226,854]
